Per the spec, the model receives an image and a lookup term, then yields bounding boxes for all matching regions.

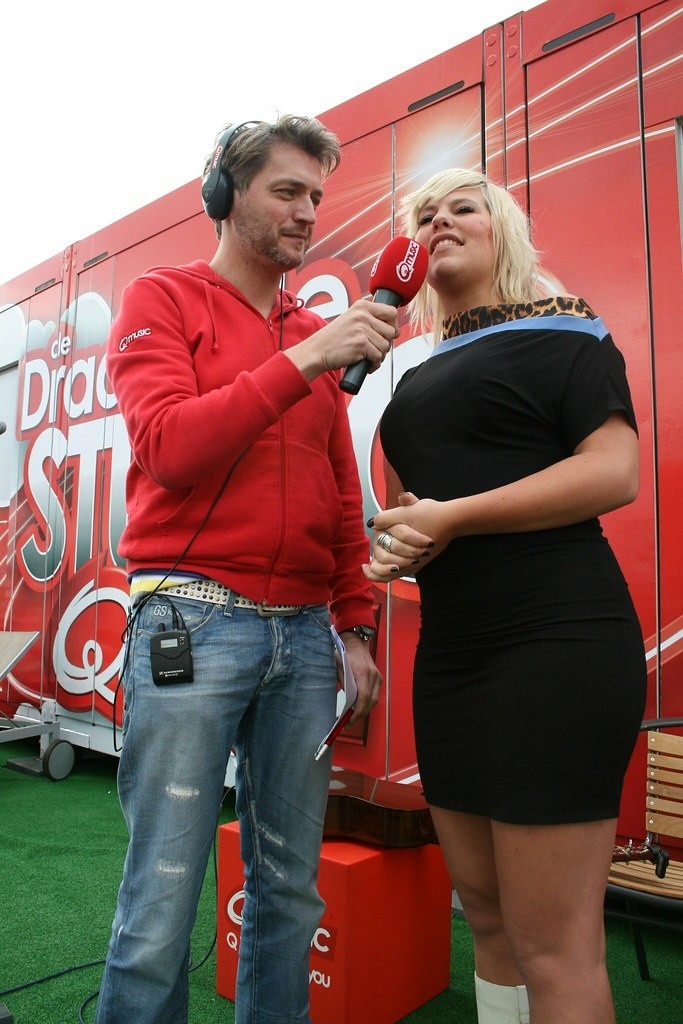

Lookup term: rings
[377,532,394,553]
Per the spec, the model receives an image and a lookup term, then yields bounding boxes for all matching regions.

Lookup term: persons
[361,169,647,1024]
[95,120,401,1024]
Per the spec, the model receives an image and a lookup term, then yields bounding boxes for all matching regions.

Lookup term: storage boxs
[218,820,452,1024]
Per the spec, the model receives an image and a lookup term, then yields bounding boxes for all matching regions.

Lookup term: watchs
[345,624,375,641]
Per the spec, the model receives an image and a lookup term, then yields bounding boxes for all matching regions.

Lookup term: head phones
[201,120,261,221]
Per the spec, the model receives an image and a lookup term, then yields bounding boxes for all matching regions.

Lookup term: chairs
[606,718,683,981]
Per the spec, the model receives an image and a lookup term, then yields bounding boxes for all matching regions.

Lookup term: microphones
[339,237,429,396]
